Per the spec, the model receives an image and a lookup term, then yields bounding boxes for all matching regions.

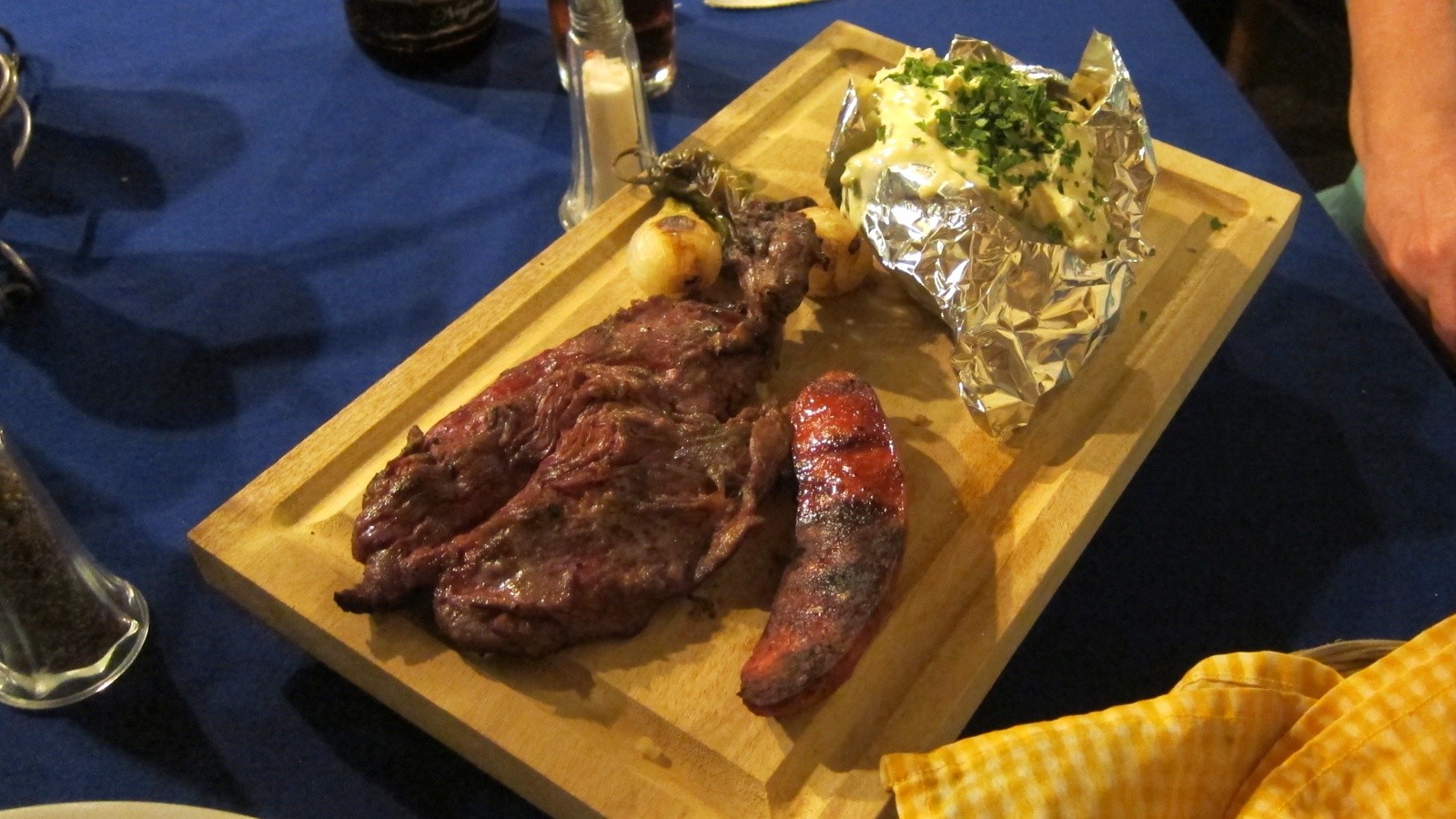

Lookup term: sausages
[743,366,900,722]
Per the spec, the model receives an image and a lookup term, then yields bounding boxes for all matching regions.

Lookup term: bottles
[346,0,501,64]
[549,1,680,100]
[551,0,658,233]
[1,425,149,714]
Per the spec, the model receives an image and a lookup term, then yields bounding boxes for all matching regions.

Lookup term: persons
[1310,2,1456,374]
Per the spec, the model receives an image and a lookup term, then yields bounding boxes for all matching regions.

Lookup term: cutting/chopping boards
[190,20,1304,817]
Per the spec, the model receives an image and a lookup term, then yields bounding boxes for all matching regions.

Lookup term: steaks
[325,283,791,675]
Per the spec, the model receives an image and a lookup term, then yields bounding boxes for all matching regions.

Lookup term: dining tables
[0,0,1456,816]
